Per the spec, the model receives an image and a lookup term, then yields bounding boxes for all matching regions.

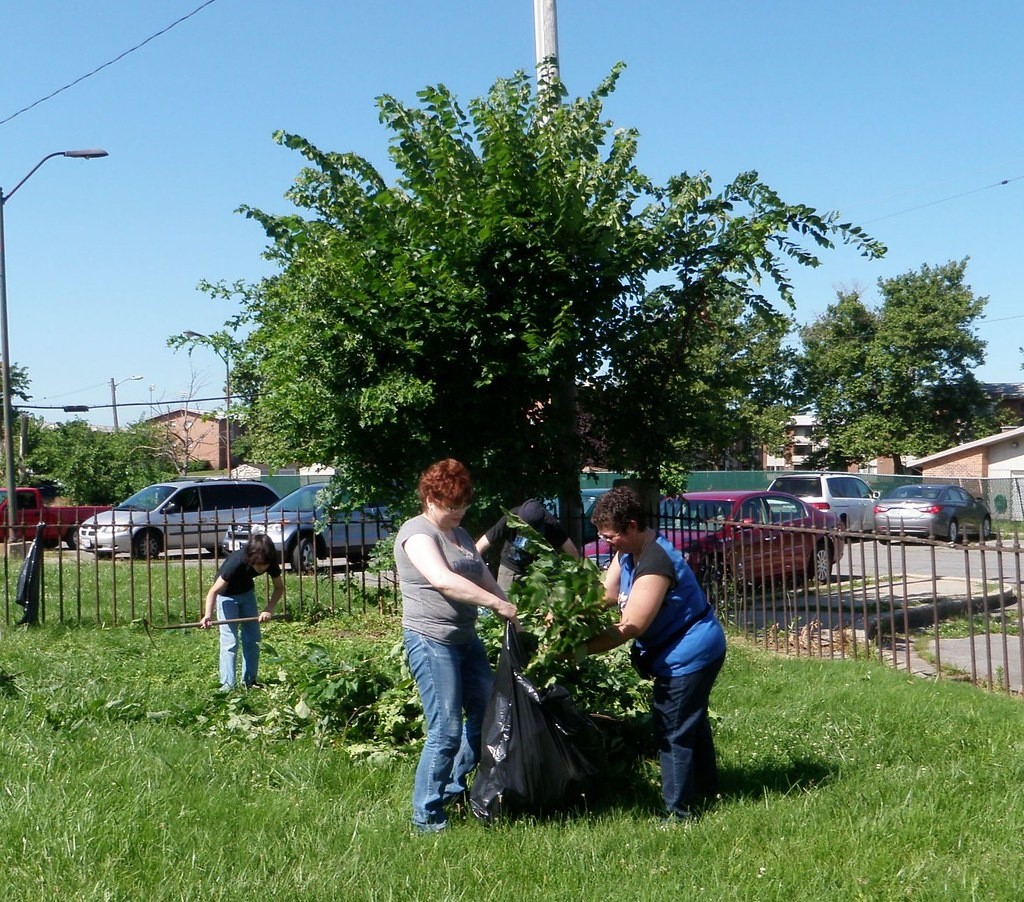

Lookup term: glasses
[430,495,473,514]
[593,527,632,543]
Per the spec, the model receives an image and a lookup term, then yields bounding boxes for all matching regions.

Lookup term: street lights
[0,147,109,543]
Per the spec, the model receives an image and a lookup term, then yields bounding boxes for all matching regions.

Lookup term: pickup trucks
[0,487,111,550]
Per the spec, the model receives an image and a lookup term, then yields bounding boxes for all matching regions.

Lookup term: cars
[767,475,880,532]
[582,489,844,597]
[873,484,992,546]
[222,482,400,573]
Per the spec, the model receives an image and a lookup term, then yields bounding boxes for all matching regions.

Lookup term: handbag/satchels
[469,611,633,826]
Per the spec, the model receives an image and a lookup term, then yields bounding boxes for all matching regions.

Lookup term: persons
[199,534,284,694]
[473,499,579,594]
[553,487,726,834]
[393,458,524,831]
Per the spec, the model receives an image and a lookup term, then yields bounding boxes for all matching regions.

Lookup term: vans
[77,479,281,560]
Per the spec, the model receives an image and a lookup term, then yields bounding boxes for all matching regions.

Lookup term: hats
[518,499,546,529]
